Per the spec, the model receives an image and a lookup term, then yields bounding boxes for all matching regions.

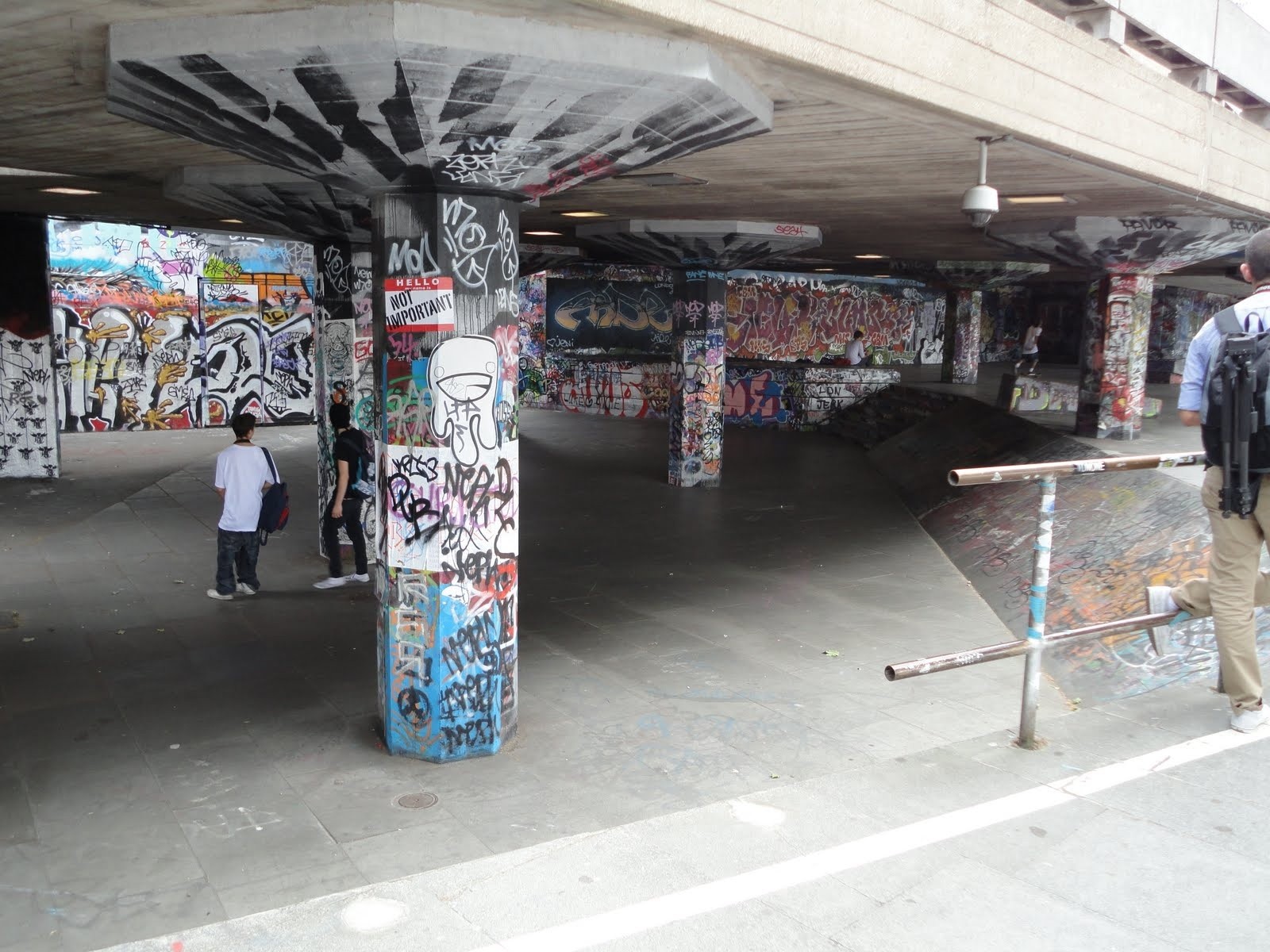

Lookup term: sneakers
[235,582,257,595]
[344,573,370,583]
[312,576,347,590]
[1230,703,1270,735]
[206,589,234,600]
[1144,585,1174,658]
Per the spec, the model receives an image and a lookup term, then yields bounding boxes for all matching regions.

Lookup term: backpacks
[334,435,376,500]
[1200,302,1270,479]
[257,447,290,546]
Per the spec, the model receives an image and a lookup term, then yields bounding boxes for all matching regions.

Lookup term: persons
[1143,226,1270,728]
[844,330,871,365]
[205,413,280,599]
[313,404,370,589]
[1014,318,1042,375]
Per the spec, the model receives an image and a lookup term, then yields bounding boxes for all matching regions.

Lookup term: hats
[329,403,351,428]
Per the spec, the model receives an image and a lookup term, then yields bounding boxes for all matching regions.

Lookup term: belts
[344,497,360,501]
